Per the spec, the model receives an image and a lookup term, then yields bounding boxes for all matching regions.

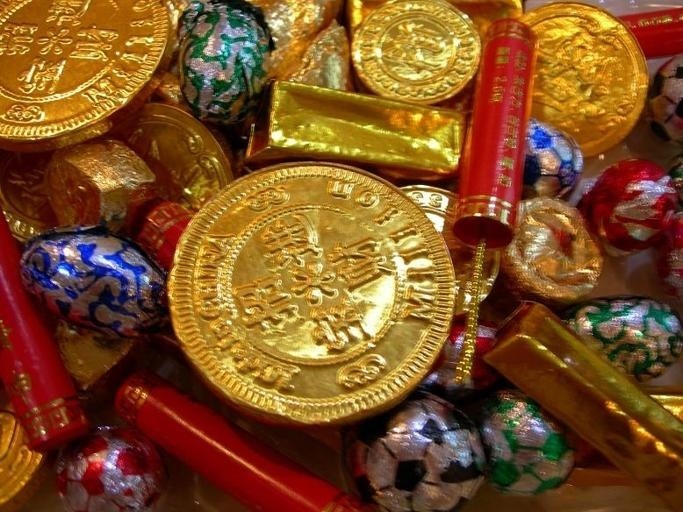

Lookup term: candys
[0,0,682,511]
